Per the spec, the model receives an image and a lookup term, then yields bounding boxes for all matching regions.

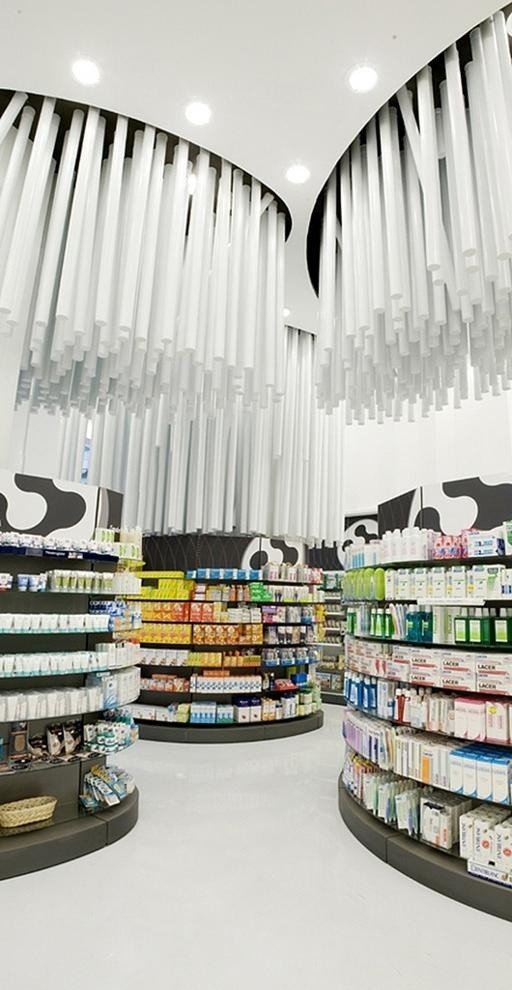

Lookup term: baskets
[0,796,57,828]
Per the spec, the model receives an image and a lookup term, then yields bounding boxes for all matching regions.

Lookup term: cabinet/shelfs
[338,471,511,923]
[1,470,140,881]
[135,531,322,746]
[305,536,346,705]
